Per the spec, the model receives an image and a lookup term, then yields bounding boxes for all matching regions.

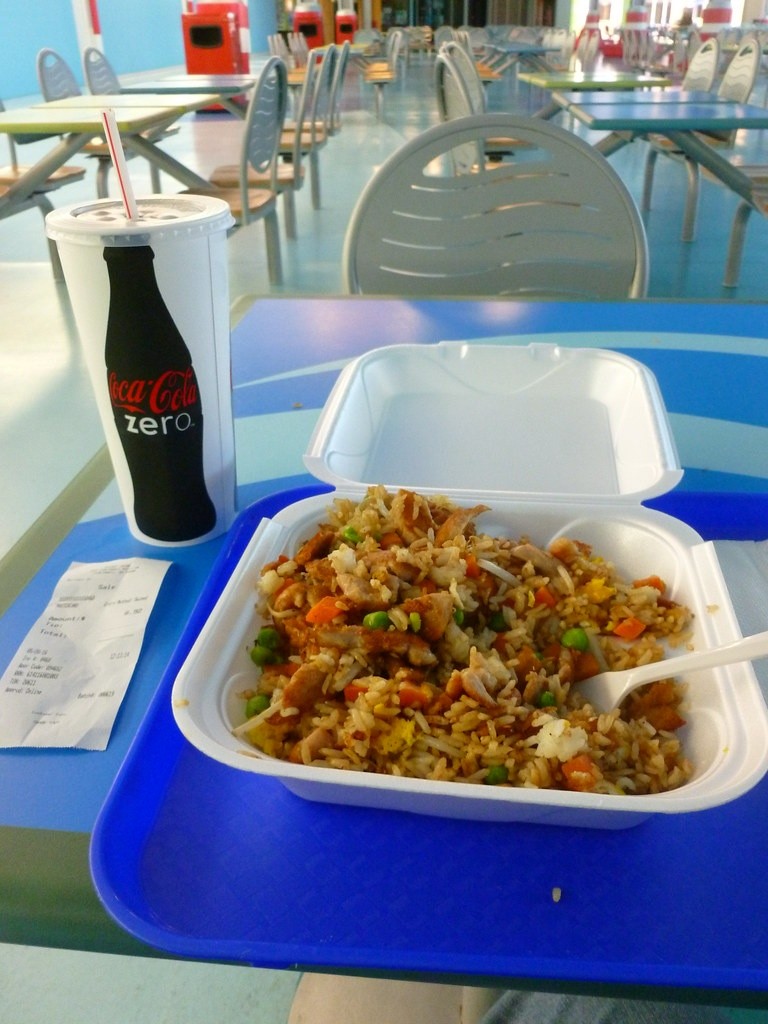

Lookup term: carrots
[262,491,691,792]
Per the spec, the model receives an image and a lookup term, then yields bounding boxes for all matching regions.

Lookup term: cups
[45,195,239,548]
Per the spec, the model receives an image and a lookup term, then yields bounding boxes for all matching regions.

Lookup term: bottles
[105,233,216,543]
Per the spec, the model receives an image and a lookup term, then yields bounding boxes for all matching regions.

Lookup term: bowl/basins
[169,338,767,829]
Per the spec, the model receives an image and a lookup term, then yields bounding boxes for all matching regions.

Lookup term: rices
[239,484,691,797]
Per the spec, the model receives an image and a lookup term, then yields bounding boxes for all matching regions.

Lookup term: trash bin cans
[181,11,241,114]
[294,16,325,67]
[336,19,355,44]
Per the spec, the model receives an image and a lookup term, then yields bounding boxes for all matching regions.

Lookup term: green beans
[246,528,588,784]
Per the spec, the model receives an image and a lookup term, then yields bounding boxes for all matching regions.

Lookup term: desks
[519,71,670,120]
[551,88,768,215]
[1,95,223,208]
[477,41,561,77]
[126,73,254,126]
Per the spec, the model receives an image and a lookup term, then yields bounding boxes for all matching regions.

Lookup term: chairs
[0,18,768,297]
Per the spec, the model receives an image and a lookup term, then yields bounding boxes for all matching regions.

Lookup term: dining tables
[0,292,768,1007]
